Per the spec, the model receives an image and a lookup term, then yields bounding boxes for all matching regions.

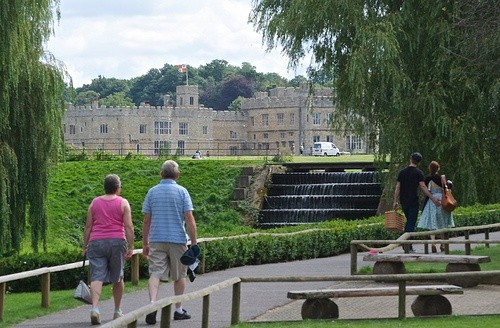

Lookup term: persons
[416,160,456,252]
[83,173,134,325]
[141,160,197,325]
[206,151,210,157]
[300,144,304,155]
[392,151,442,251]
[291,143,296,154]
[192,150,202,158]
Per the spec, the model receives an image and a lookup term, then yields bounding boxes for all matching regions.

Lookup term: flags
[177,64,186,73]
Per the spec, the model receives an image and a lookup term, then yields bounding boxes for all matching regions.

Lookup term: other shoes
[90,310,102,325]
[440,247,445,253]
[432,246,437,253]
[113,312,123,320]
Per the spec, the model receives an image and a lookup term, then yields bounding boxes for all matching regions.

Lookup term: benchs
[363,254,491,288]
[287,285,463,320]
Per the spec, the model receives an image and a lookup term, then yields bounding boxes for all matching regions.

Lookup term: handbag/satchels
[74,280,91,305]
[442,175,458,211]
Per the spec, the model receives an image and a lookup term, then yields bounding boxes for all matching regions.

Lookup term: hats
[180,244,200,265]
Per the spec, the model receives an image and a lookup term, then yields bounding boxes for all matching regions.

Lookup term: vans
[312,142,339,156]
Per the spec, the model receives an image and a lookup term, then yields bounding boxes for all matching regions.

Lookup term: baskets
[385,209,404,232]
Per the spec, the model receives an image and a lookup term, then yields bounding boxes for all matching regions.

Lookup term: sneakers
[145,310,157,325]
[174,308,191,320]
